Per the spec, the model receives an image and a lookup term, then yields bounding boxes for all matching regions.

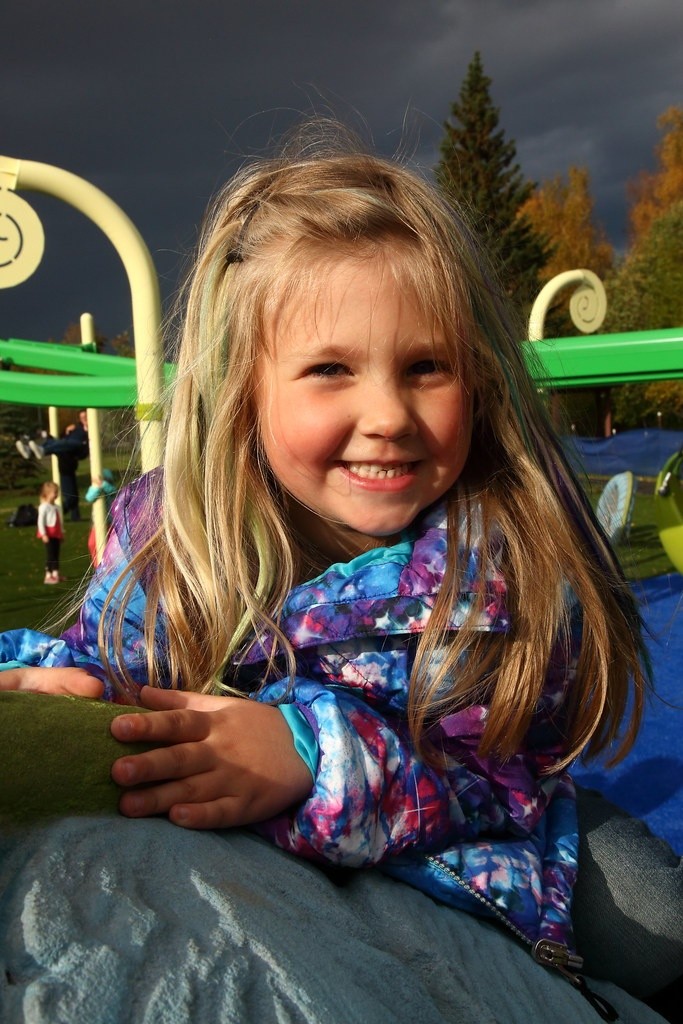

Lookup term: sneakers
[16,440,32,460]
[29,440,45,460]
[44,575,67,584]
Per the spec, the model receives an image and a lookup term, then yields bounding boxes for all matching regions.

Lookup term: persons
[0,148,651,953]
[36,480,66,585]
[54,425,82,520]
[14,410,92,462]
